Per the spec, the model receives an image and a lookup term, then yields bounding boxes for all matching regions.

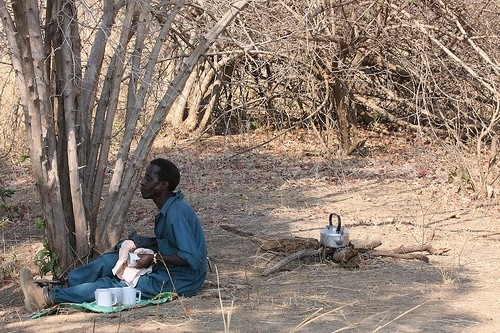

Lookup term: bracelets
[153,252,157,265]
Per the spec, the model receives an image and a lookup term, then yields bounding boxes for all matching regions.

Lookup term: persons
[20,157,207,313]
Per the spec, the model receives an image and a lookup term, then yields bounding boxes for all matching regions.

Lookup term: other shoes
[30,279,69,290]
[20,268,52,313]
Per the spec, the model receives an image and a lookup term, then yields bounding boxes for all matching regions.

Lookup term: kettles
[320,213,349,248]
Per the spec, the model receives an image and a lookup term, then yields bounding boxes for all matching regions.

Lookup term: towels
[112,240,155,289]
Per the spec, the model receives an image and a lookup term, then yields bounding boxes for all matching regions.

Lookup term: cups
[94,287,141,310]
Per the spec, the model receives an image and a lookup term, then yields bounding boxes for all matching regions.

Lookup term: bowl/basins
[136,254,154,268]
